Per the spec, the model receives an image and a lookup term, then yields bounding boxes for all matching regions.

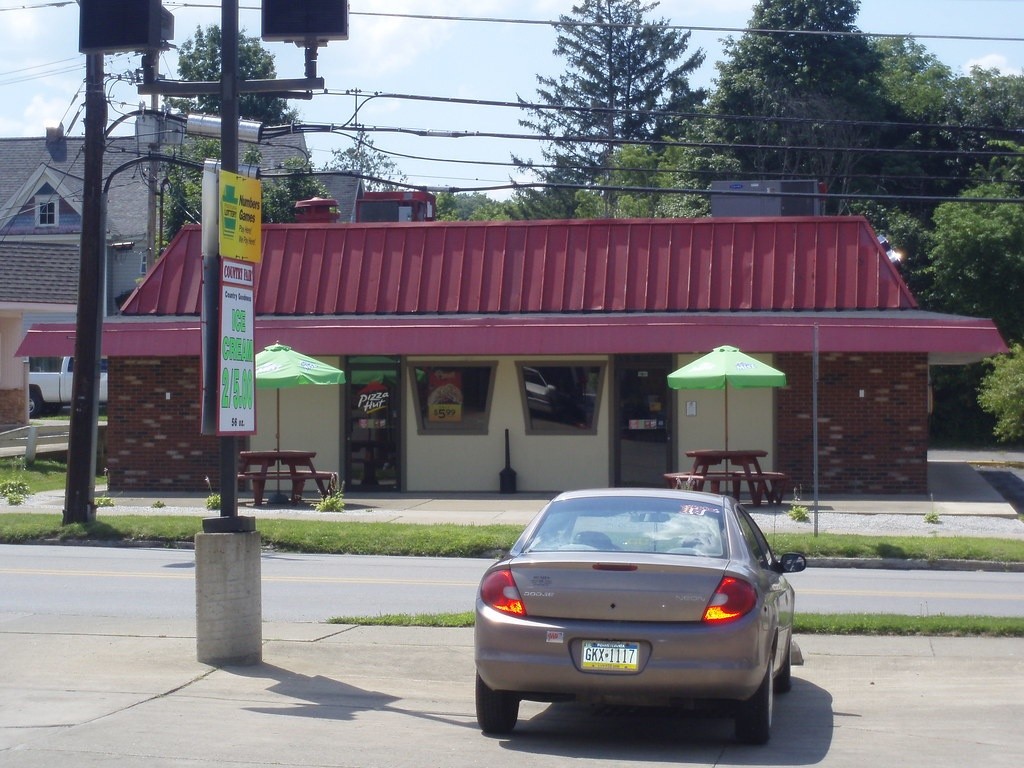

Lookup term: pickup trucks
[29,355,108,417]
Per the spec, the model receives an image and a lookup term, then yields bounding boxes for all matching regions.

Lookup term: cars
[474,487,807,747]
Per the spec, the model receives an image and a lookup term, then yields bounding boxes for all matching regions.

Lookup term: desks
[686,450,771,505]
[241,451,328,506]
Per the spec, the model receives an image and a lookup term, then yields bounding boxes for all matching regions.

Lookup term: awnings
[14,318,1007,354]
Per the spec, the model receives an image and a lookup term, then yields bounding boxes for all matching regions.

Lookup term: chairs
[558,532,612,551]
[667,532,722,556]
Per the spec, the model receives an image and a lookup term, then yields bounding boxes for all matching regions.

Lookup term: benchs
[664,471,786,505]
[238,471,333,505]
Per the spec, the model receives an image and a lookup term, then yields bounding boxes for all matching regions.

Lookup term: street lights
[76,2,352,536]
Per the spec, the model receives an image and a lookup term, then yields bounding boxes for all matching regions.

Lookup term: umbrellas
[342,354,426,444]
[253,342,347,506]
[666,345,786,496]
